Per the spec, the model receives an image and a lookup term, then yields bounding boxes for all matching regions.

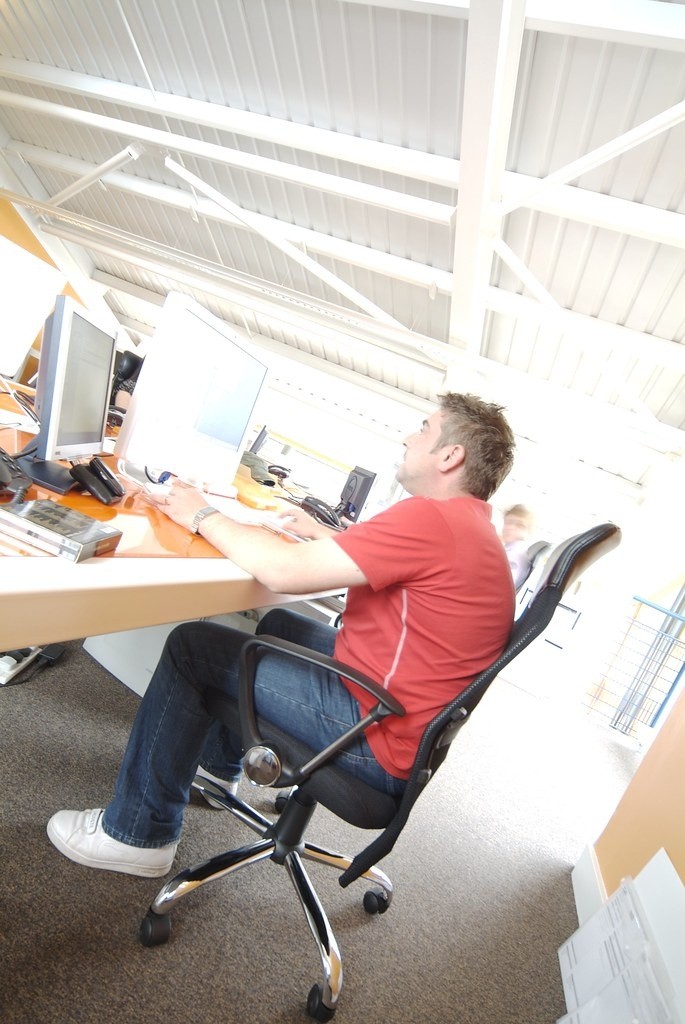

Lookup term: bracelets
[190,507,220,537]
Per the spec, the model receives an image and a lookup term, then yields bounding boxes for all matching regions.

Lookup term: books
[0,499,123,564]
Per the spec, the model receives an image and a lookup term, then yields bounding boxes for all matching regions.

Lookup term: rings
[165,496,169,504]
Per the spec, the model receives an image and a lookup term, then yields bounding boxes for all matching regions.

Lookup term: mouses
[263,511,298,537]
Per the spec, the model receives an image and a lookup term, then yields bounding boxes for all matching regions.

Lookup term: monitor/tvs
[17,291,275,497]
[334,466,379,529]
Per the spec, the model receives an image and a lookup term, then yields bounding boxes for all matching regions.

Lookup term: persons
[501,505,534,589]
[47,393,516,877]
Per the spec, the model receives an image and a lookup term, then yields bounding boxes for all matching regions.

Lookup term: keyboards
[143,482,264,527]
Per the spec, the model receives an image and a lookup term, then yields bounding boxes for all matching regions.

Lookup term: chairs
[137,523,619,1020]
[514,539,552,593]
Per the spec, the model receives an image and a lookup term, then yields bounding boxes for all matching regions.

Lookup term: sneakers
[190,765,238,810]
[47,808,181,878]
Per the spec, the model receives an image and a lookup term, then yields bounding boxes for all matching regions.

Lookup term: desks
[1,375,350,649]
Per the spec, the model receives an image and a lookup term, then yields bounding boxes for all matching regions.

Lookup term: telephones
[268,465,291,479]
[68,460,113,505]
[0,446,33,495]
[302,496,343,528]
